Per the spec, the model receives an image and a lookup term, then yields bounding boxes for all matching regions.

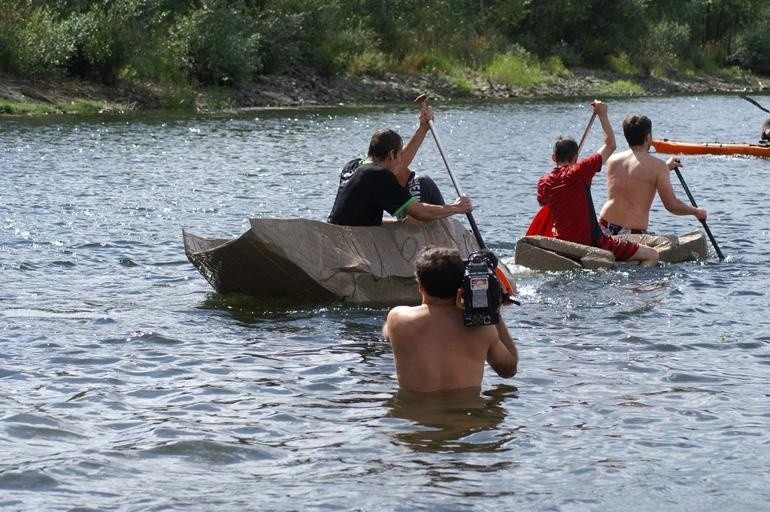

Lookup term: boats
[515,229,708,272]
[182,216,517,303]
[651,139,770,157]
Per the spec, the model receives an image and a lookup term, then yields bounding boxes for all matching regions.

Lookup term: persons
[595,112,708,238]
[394,167,447,222]
[326,106,475,226]
[382,243,519,397]
[537,99,661,268]
[759,117,770,147]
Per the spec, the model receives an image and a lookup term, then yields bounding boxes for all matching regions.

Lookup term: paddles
[525,100,597,238]
[414,89,514,297]
